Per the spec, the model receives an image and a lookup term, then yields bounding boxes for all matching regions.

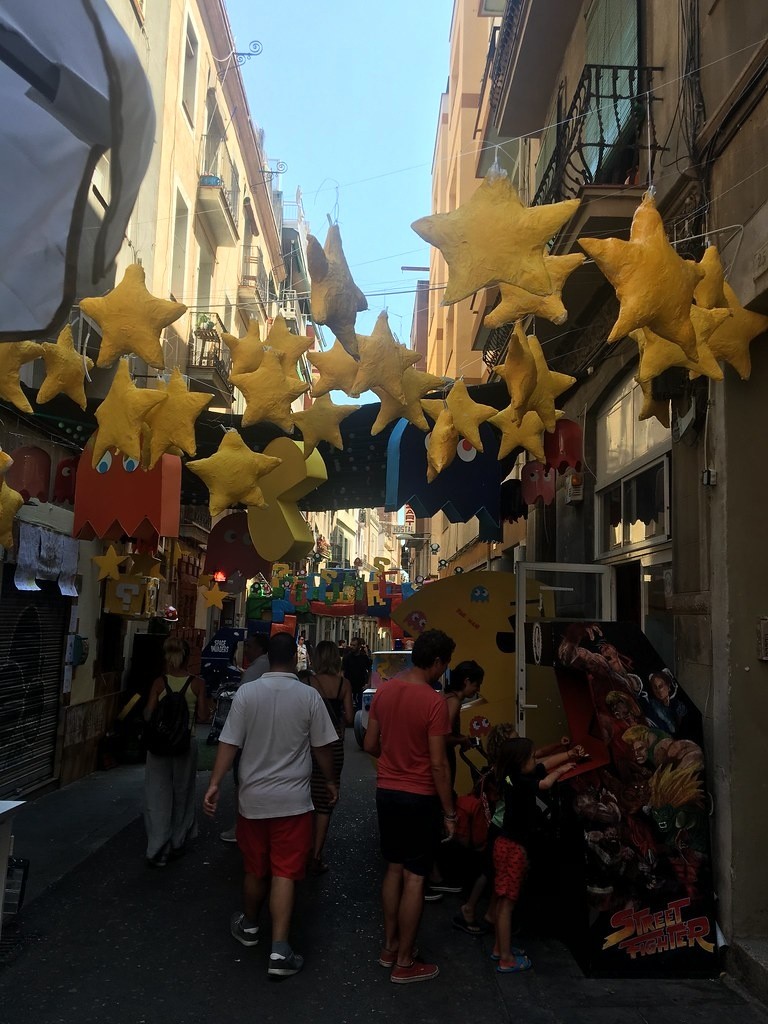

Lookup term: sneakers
[388,963,439,983]
[377,946,419,966]
[229,909,259,946]
[266,953,305,977]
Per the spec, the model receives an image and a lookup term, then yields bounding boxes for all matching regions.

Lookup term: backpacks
[147,673,195,756]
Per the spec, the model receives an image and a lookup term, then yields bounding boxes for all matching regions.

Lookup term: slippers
[450,916,486,935]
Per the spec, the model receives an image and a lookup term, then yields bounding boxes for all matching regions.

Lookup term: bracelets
[567,751,570,759]
[444,810,458,823]
[326,780,334,784]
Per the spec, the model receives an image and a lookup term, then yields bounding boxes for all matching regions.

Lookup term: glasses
[301,639,304,641]
[350,643,356,647]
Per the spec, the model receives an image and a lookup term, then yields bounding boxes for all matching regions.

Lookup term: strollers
[206,675,240,743]
[458,740,490,784]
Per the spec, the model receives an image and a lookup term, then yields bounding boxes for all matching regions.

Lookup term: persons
[220,634,372,877]
[452,722,585,971]
[142,637,209,866]
[201,631,339,976]
[423,661,484,901]
[364,628,458,985]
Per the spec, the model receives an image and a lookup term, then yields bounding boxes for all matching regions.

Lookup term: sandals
[497,956,535,973]
[492,947,528,960]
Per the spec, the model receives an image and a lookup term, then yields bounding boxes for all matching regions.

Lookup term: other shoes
[219,824,237,841]
[424,890,443,901]
[310,859,329,876]
[429,879,463,892]
[144,861,168,869]
[171,837,192,860]
